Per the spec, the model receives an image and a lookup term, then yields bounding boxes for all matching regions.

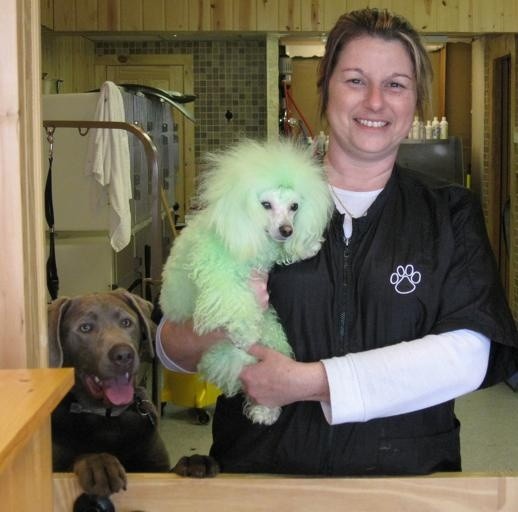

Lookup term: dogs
[47,286,220,499]
[159,136,335,426]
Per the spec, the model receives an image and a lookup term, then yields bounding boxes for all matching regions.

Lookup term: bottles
[439,116,448,139]
[432,116,439,140]
[419,121,426,140]
[412,116,420,140]
[424,120,432,140]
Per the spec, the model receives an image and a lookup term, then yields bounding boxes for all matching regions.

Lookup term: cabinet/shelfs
[40,93,175,401]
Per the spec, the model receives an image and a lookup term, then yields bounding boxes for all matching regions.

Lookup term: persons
[155,7,518,479]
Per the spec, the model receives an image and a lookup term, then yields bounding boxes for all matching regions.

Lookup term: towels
[92,78,135,253]
